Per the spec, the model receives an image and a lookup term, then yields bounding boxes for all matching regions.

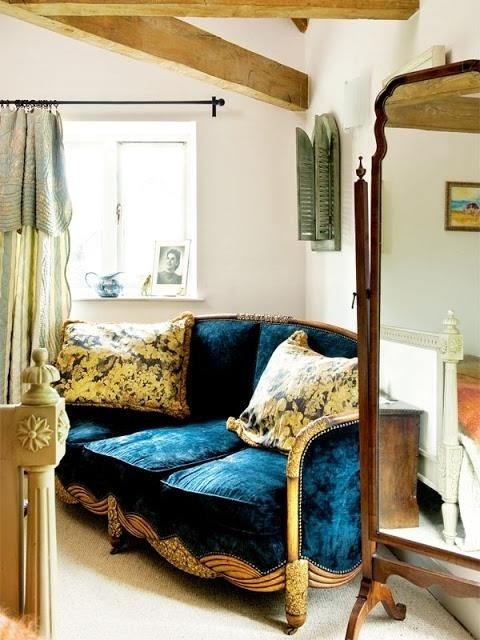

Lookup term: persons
[156,247,182,285]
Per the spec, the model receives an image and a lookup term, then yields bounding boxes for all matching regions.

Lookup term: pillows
[224,330,360,458]
[54,314,193,420]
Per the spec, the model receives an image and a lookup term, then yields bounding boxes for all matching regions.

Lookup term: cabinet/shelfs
[377,392,423,529]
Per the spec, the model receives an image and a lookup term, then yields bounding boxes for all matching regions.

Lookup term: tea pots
[84,271,126,298]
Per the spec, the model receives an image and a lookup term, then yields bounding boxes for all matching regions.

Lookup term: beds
[379,308,480,554]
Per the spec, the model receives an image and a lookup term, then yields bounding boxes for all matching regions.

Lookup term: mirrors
[345,58,480,640]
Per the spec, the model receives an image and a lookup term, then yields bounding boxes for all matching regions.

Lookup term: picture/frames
[446,181,480,231]
[153,240,188,295]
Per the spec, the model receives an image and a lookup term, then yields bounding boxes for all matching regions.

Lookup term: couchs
[49,313,364,635]
